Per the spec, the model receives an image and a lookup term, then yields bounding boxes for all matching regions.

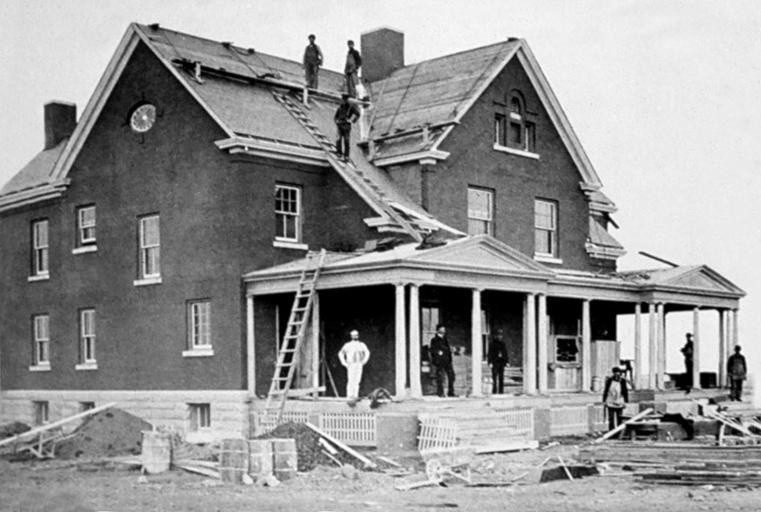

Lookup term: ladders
[258,248,326,437]
[269,85,438,244]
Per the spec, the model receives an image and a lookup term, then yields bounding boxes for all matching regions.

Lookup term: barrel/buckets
[220,438,248,482]
[274,439,297,480]
[141,431,170,472]
[250,439,273,478]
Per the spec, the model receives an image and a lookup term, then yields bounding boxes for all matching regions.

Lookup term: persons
[337,330,370,404]
[345,40,362,98]
[680,332,693,387]
[487,330,510,394]
[303,34,323,89]
[727,345,746,401]
[430,323,459,397]
[604,367,628,438]
[334,94,361,161]
